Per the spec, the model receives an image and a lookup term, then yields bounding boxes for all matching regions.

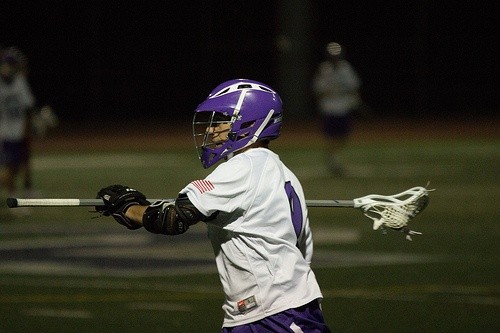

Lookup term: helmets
[192,79,285,169]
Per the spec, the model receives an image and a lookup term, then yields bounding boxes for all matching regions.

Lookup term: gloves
[94,184,151,231]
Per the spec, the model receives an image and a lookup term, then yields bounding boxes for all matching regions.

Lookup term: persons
[317,44,359,177]
[0,50,36,193]
[92,79,326,333]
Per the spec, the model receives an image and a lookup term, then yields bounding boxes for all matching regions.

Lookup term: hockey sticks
[7,186,437,242]
